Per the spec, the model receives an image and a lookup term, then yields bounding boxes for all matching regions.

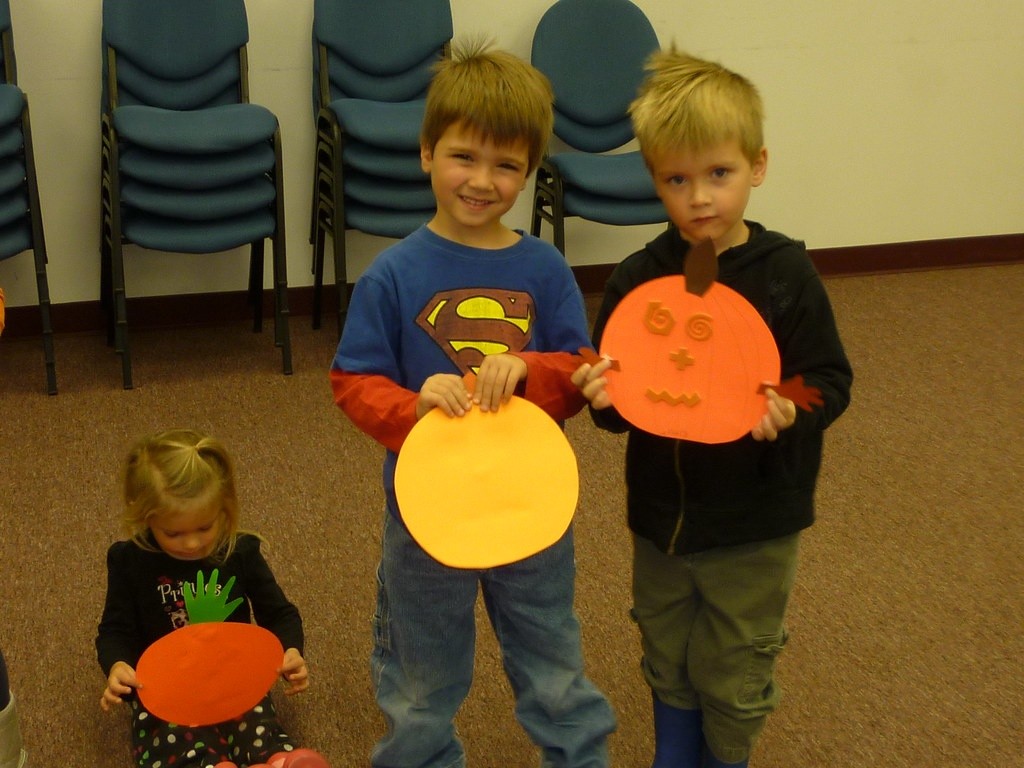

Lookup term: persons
[328,34,621,767]
[94,427,326,768]
[572,44,855,768]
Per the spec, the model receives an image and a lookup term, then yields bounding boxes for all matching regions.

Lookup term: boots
[650,693,705,768]
[706,746,748,768]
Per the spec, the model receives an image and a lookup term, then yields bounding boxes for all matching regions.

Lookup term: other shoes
[216,749,329,768]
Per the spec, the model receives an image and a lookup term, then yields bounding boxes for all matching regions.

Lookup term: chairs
[99,0,293,390]
[530,0,674,257]
[307,0,452,342]
[0,0,56,395]
